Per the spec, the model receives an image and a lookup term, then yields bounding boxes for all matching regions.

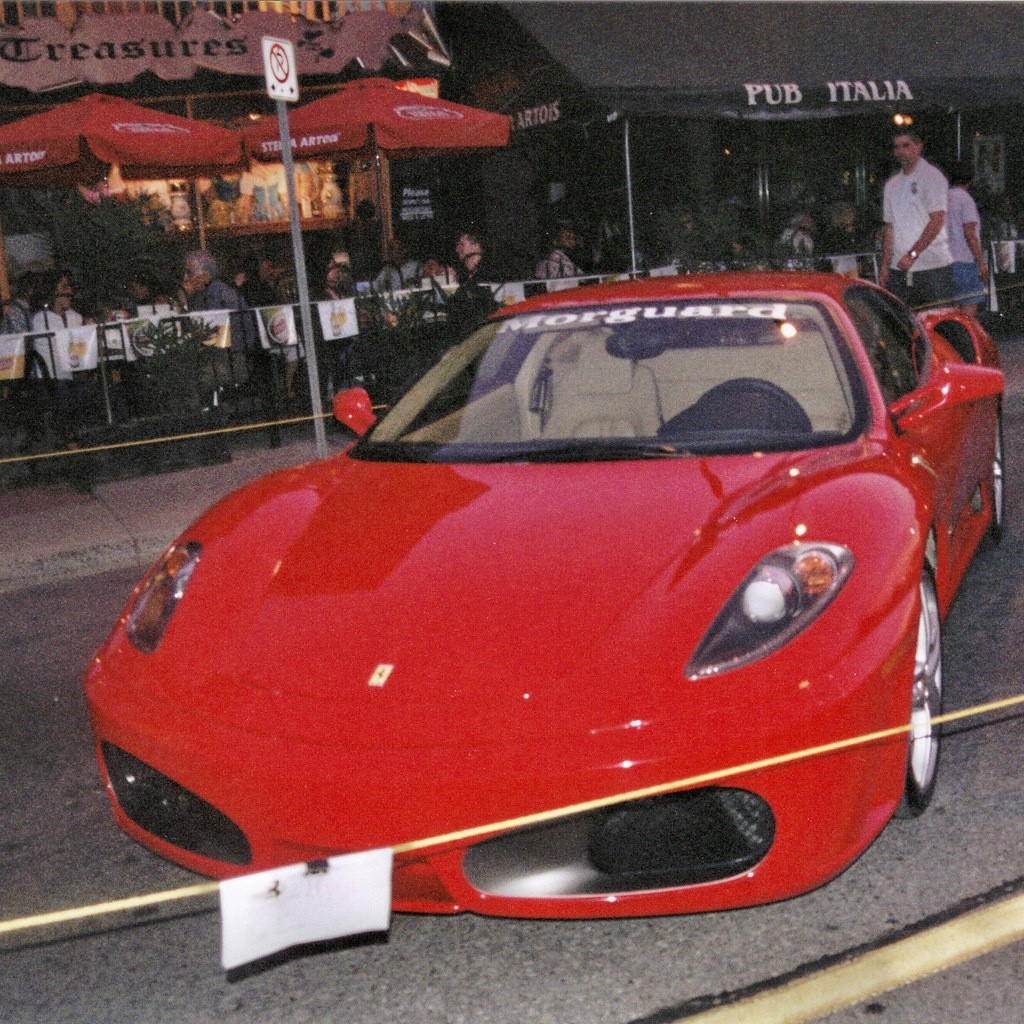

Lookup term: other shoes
[200,451,232,466]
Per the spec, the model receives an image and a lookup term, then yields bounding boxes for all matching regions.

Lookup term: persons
[880,128,970,361]
[946,161,987,358]
[3,196,1021,456]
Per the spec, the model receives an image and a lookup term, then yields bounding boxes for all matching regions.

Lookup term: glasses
[56,292,75,299]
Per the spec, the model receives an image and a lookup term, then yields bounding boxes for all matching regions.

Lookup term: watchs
[908,250,918,260]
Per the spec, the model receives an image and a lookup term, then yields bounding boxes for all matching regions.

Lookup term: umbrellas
[2,78,513,254]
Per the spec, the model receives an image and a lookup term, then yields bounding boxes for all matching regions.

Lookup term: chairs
[537,335,663,438]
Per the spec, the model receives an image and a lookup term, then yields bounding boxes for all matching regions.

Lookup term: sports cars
[80,270,1005,922]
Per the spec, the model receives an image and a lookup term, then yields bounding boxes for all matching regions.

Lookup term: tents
[500,0,1023,276]
